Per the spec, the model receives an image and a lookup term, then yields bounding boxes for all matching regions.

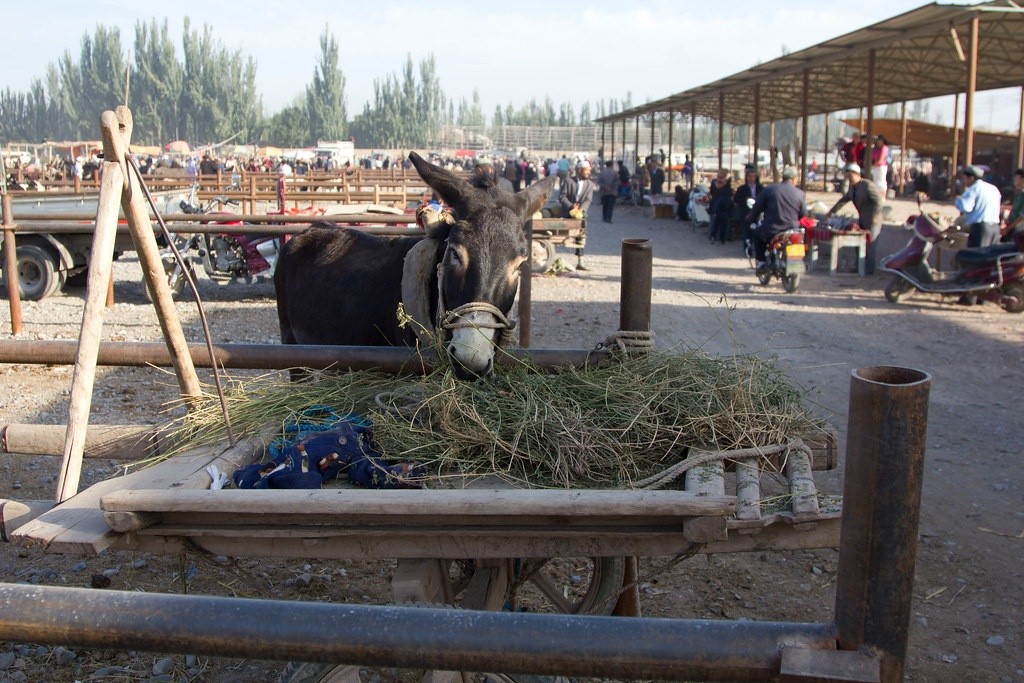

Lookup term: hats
[845,162,861,174]
[781,166,798,178]
[965,165,985,179]
[474,158,492,167]
[576,160,591,168]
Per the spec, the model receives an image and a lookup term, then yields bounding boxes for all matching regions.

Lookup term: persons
[911,161,1024,306]
[706,168,734,246]
[807,131,890,273]
[674,185,692,221]
[540,151,594,183]
[744,166,807,273]
[58,153,102,181]
[616,160,630,199]
[225,154,309,174]
[495,148,547,192]
[681,154,692,189]
[559,161,594,271]
[734,163,767,256]
[598,160,620,223]
[309,153,339,172]
[659,149,666,164]
[423,152,493,170]
[648,160,665,194]
[353,153,413,170]
[130,152,224,190]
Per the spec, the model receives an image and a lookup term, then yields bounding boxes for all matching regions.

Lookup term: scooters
[879,190,1024,315]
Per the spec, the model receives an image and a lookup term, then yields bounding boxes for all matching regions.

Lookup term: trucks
[0,181,199,302]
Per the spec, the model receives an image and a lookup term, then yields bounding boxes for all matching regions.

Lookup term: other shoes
[575,264,590,271]
[756,264,768,277]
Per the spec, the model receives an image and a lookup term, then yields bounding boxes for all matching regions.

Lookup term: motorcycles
[743,196,807,292]
[140,180,328,304]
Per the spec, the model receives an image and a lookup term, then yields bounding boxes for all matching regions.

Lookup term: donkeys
[275,151,560,381]
